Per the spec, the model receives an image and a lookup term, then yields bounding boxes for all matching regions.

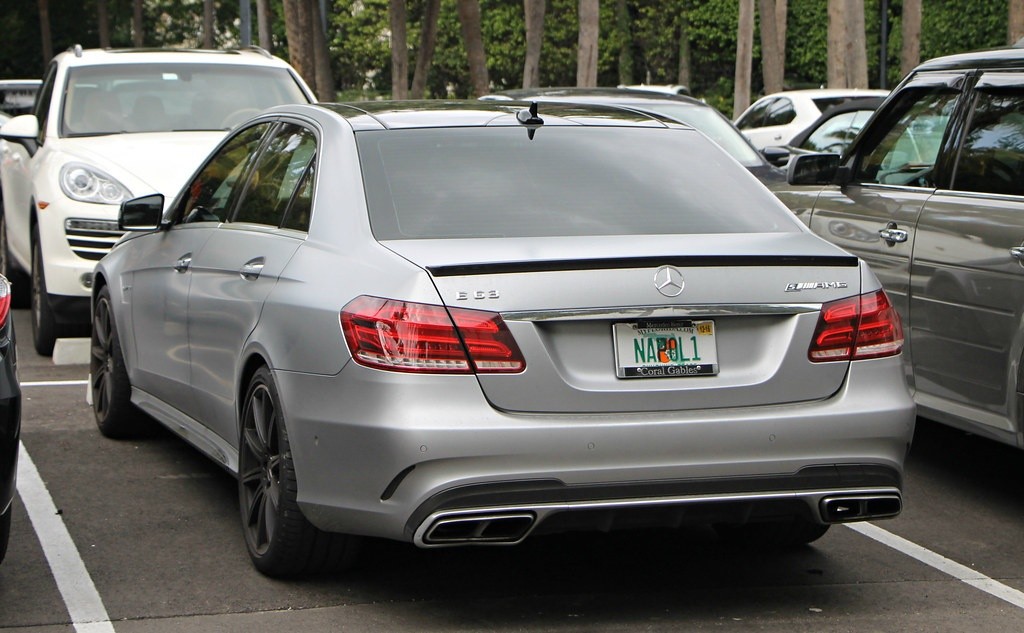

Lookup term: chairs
[73,90,257,130]
[960,115,1024,193]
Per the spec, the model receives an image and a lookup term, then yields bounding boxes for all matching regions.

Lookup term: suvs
[0,43,324,357]
[765,36,1024,450]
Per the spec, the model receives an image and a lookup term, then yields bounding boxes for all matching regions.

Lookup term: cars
[483,84,790,186]
[733,88,890,148]
[787,98,951,180]
[0,79,95,134]
[89,99,917,579]
[0,212,23,560]
